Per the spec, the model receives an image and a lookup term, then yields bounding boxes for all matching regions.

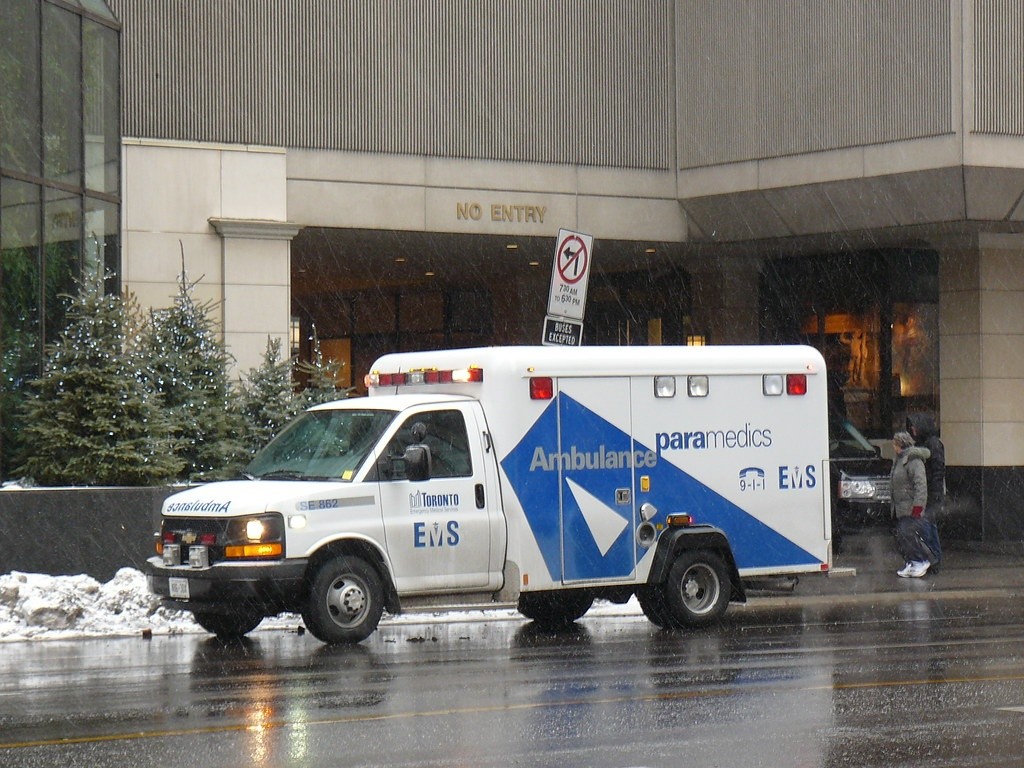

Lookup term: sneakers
[911,561,929,578]
[899,559,916,577]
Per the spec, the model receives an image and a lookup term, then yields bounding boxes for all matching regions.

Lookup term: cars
[828,397,894,529]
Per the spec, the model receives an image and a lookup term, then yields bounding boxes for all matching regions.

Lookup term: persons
[911,413,946,574]
[889,431,930,577]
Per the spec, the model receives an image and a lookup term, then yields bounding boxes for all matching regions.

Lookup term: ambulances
[140,342,857,647]
